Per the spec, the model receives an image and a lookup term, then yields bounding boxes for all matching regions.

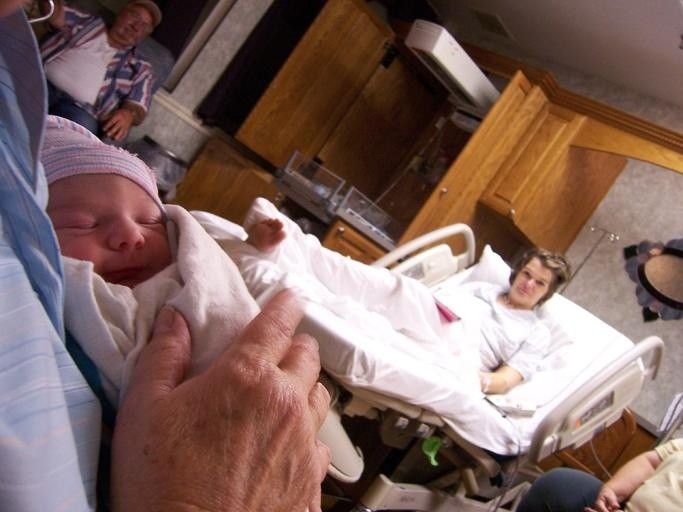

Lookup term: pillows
[435,246,598,413]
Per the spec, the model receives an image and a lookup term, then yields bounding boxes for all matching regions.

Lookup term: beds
[190,222,663,512]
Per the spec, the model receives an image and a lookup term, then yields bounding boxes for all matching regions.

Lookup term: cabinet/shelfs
[175,0,628,261]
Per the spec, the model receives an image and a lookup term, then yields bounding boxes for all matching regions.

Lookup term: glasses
[126,7,153,33]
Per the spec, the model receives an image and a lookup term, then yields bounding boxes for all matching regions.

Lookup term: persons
[511,435,682,512]
[0,0,331,512]
[30,110,269,402]
[34,0,161,142]
[247,196,566,394]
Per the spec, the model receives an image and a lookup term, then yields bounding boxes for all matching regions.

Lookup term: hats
[41,115,169,223]
[130,0,161,27]
